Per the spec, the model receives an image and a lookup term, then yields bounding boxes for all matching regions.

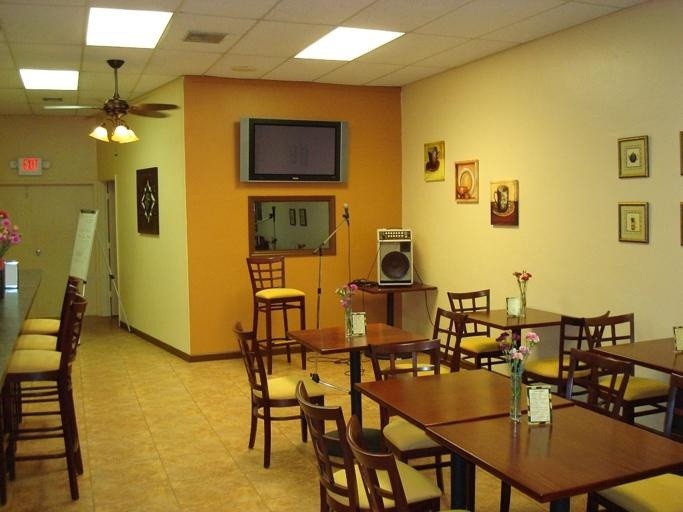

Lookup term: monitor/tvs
[240,117,348,183]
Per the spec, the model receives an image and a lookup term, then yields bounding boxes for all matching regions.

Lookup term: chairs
[0,275,86,506]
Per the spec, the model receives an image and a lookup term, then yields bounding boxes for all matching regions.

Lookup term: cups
[456,186,468,199]
[494,185,510,210]
[427,146,441,166]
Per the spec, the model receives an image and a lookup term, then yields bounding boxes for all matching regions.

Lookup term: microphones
[272,206,276,222]
[344,203,349,223]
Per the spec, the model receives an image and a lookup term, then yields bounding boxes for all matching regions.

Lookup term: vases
[135,167,159,235]
[0,256,6,299]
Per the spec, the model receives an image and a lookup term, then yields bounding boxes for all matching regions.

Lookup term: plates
[426,159,441,172]
[492,201,515,218]
[458,168,475,198]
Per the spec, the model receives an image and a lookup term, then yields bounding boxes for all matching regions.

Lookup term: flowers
[0,209,22,257]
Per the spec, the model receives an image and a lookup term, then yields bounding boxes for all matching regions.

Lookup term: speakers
[377,228,413,286]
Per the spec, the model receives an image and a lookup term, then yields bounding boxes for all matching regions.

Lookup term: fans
[42,59,179,118]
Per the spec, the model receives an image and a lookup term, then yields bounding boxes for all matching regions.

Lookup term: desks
[0,269,43,394]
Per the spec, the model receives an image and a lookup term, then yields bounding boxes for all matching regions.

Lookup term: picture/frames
[454,158,478,203]
[299,208,307,225]
[488,179,519,225]
[616,202,648,243]
[289,208,296,225]
[679,131,683,176]
[422,141,444,182]
[680,202,683,245]
[616,135,648,179]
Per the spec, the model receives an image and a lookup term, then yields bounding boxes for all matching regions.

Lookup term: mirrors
[247,195,337,257]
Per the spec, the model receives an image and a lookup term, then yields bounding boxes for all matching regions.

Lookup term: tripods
[308,220,353,394]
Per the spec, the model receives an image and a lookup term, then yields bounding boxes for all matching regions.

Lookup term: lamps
[87,118,140,144]
